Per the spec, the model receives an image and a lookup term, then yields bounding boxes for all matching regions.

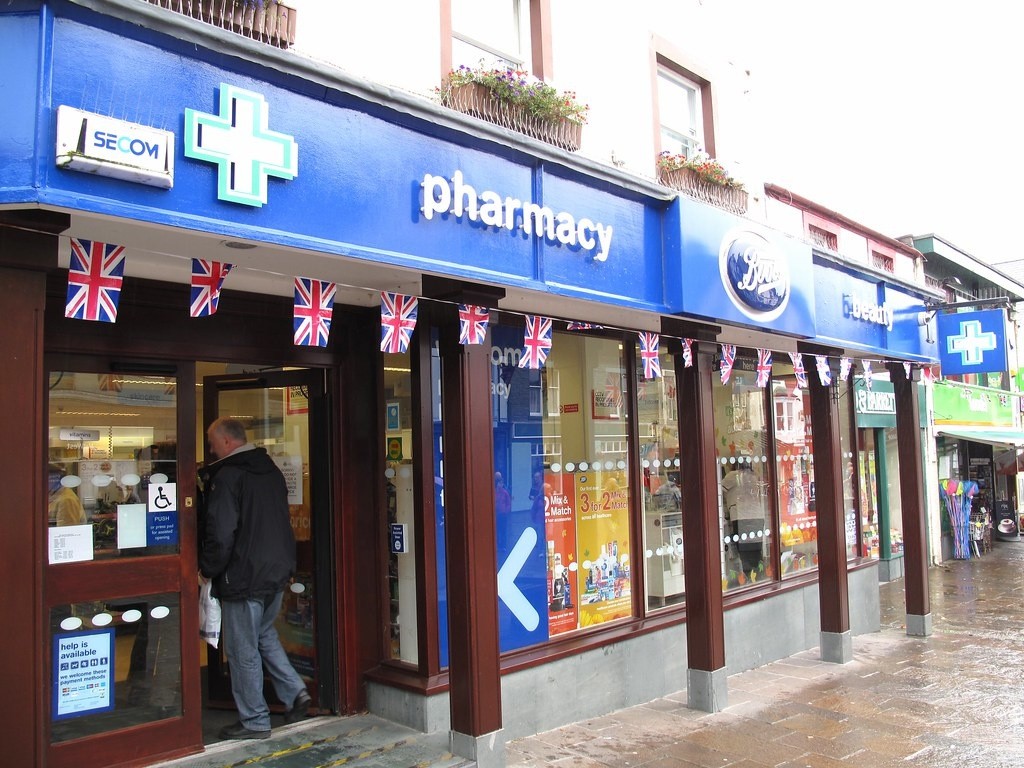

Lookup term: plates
[998,524,1016,533]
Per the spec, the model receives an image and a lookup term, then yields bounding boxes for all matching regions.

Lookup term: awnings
[936,425,1023,449]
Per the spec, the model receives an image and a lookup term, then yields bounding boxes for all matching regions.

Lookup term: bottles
[550,553,564,611]
[788,446,816,514]
[547,540,555,605]
[673,451,680,470]
[587,542,622,599]
[564,567,570,607]
[645,487,652,511]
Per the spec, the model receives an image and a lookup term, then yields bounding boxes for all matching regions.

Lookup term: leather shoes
[286,691,312,721]
[220,722,272,739]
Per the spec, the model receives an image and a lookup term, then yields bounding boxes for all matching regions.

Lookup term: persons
[124,441,201,717]
[721,458,765,579]
[494,471,512,553]
[527,472,557,558]
[655,475,681,511]
[198,417,312,741]
[47,460,87,525]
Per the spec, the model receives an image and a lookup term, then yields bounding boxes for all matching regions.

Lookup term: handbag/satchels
[198,578,222,648]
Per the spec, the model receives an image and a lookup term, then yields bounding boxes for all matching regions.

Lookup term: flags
[754,348,773,388]
[379,292,418,354]
[840,356,854,381]
[787,352,807,389]
[719,344,736,386]
[638,332,661,378]
[457,303,489,345]
[292,277,337,347]
[681,338,696,368]
[903,361,911,380]
[862,359,873,391]
[518,315,552,370]
[189,258,232,318]
[65,236,127,323]
[567,321,603,330]
[815,354,832,387]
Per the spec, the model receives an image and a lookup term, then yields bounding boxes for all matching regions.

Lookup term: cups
[1000,519,1014,530]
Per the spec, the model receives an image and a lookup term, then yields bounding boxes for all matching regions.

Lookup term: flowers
[656,146,743,189]
[236,0,287,18]
[428,57,590,127]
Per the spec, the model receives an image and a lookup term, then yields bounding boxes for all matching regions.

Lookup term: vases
[172,0,296,50]
[659,168,750,217]
[450,81,582,152]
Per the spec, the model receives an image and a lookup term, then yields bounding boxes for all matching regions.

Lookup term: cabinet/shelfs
[646,511,685,607]
